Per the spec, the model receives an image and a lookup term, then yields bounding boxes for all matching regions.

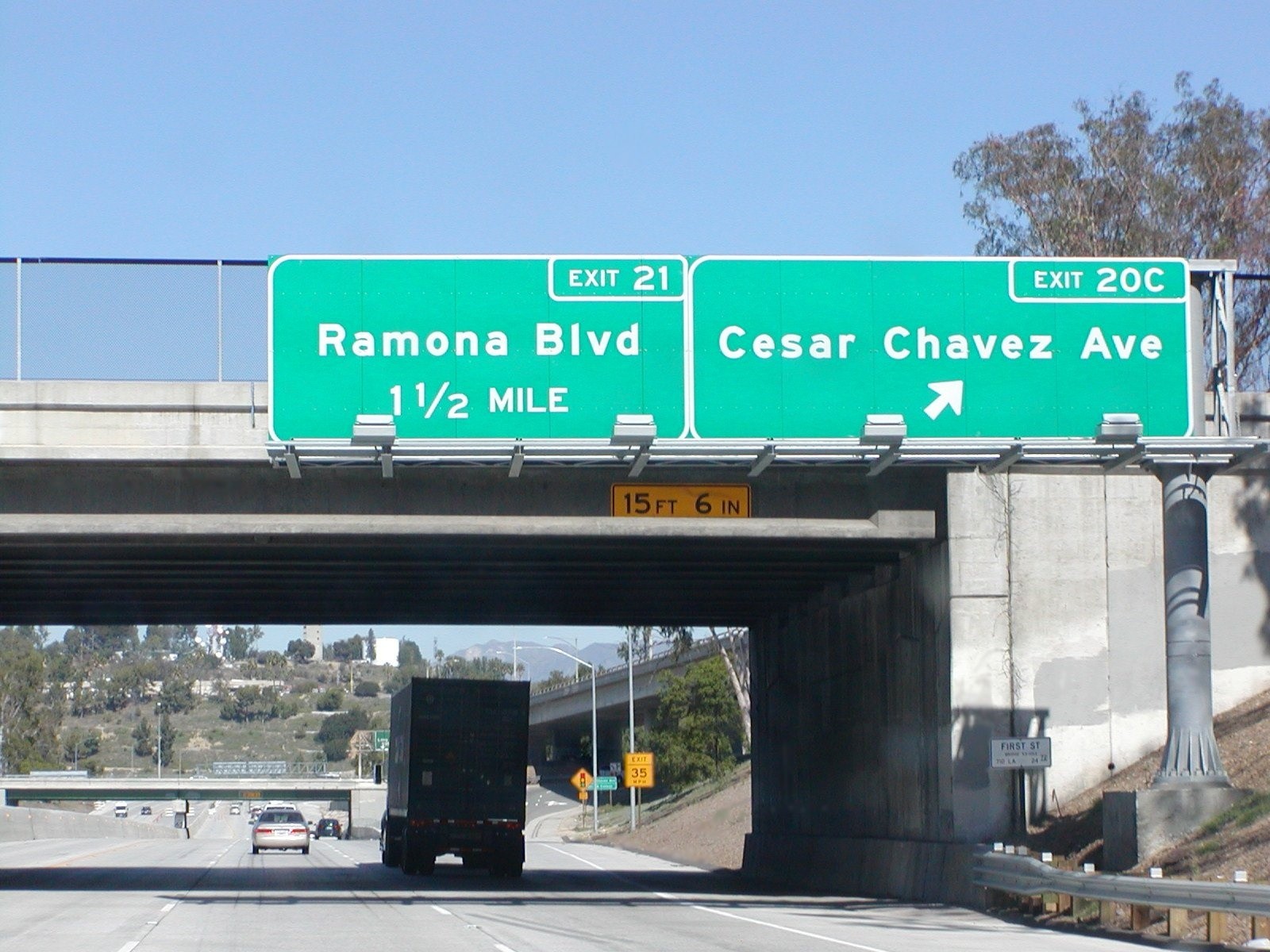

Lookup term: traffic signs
[689,254,1194,442]
[268,255,687,446]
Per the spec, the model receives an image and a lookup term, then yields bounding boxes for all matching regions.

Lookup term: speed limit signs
[623,753,653,788]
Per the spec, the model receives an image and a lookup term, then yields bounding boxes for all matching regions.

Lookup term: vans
[114,802,128,817]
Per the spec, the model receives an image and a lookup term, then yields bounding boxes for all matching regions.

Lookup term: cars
[141,806,151,815]
[248,803,296,823]
[228,801,243,815]
[249,810,313,854]
[313,819,343,840]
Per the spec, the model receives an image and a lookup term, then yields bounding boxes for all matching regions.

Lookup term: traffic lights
[581,773,586,787]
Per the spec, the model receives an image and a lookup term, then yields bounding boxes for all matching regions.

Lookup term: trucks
[372,676,530,876]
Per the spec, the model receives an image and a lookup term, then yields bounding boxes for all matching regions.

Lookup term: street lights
[543,635,578,684]
[156,702,162,779]
[496,650,530,682]
[514,645,598,834]
[75,737,99,770]
